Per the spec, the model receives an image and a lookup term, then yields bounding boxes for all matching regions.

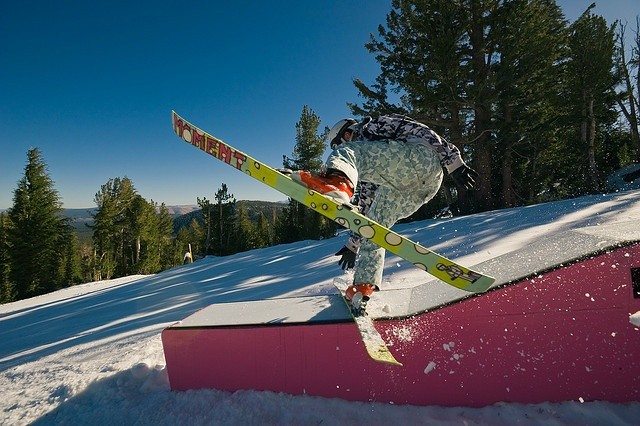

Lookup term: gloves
[335,247,356,270]
[449,164,479,190]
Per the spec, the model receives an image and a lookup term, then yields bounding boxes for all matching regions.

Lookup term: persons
[291,115,480,311]
[183,249,191,265]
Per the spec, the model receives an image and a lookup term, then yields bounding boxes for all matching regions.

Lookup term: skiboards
[172,110,496,366]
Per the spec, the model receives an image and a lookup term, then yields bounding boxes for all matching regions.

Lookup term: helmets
[330,118,357,150]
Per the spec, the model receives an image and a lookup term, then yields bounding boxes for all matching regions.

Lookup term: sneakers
[291,170,354,206]
[345,284,375,302]
